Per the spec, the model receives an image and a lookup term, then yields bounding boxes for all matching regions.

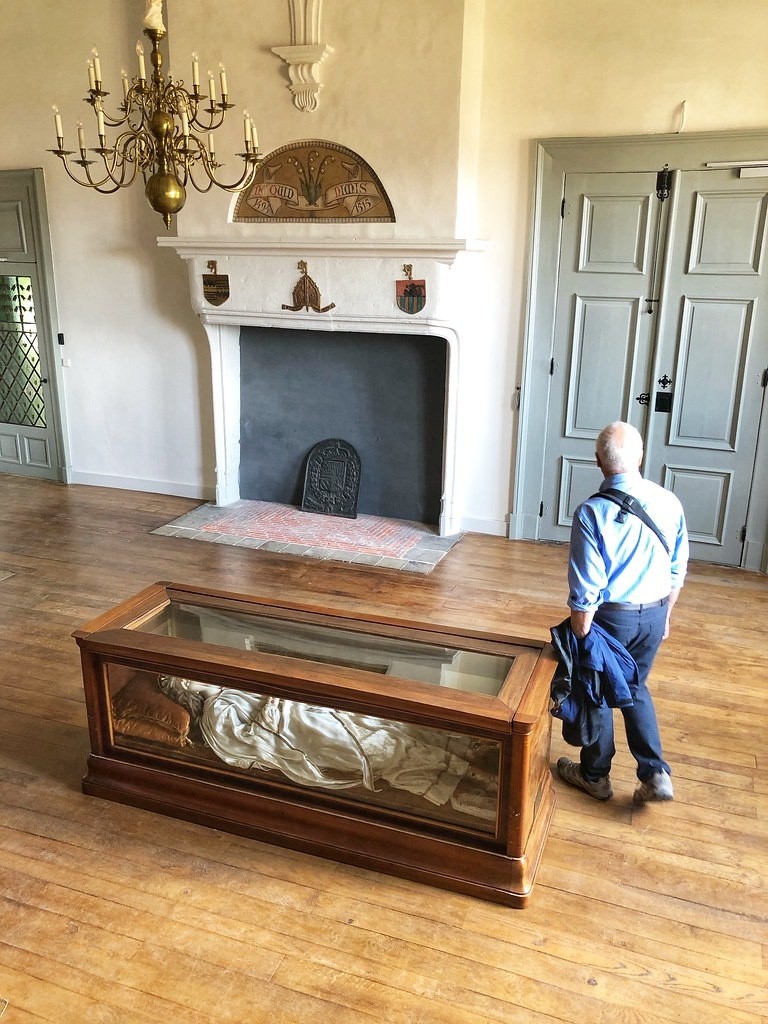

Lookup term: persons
[556,420,691,808]
[158,673,501,792]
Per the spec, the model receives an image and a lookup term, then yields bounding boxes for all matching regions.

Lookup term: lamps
[46,0,263,230]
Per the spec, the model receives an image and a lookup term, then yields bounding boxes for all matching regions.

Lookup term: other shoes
[557,756,614,799]
[633,770,674,803]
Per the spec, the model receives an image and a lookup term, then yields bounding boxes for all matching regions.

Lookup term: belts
[600,595,670,611]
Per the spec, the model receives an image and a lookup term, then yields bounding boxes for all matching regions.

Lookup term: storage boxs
[70,580,557,908]
